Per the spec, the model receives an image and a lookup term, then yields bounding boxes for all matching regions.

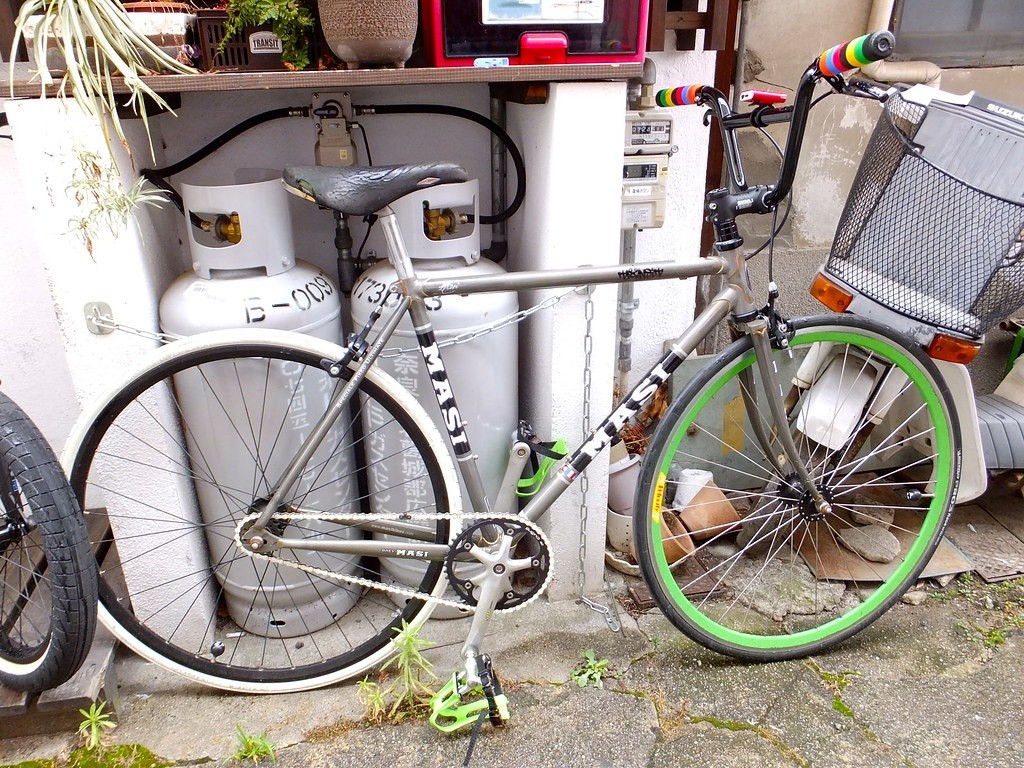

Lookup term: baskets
[825,92,1024,337]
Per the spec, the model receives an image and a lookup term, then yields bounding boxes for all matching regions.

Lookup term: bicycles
[59,30,967,740]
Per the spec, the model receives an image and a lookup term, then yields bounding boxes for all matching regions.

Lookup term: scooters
[734,60,1023,559]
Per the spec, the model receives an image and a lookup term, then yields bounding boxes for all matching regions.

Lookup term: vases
[610,438,630,468]
[671,468,714,513]
[631,505,696,565]
[679,480,743,540]
[316,0,418,70]
[122,2,191,48]
[608,453,642,516]
[607,506,632,553]
[605,542,643,577]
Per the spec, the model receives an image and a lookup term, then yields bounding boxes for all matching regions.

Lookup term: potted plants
[196,0,314,74]
[12,0,104,79]
